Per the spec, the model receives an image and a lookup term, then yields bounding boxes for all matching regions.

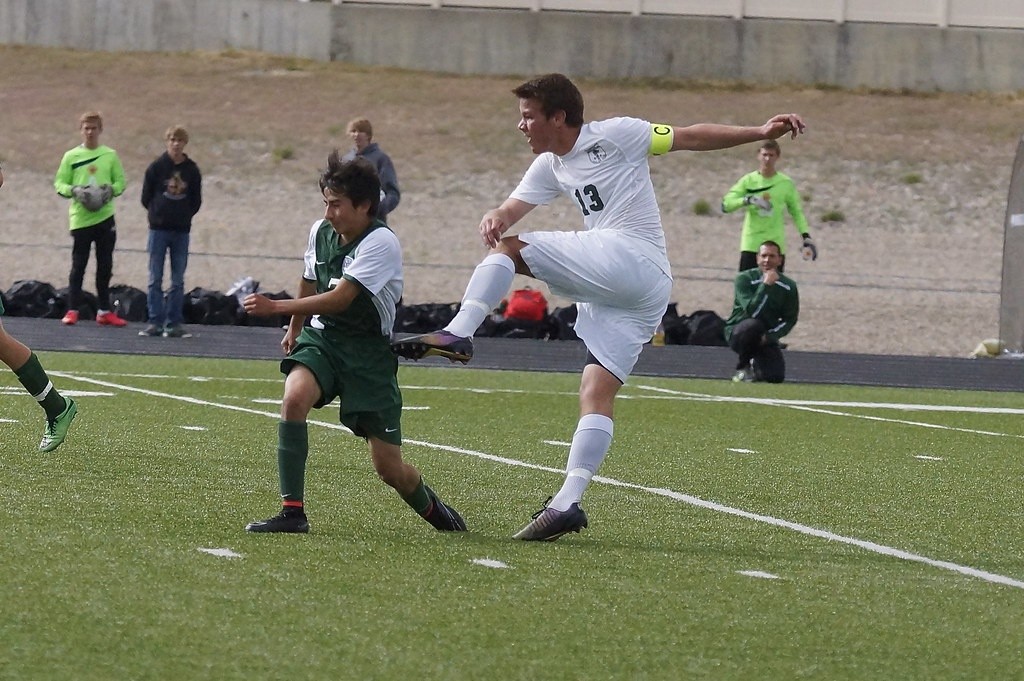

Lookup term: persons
[242,152,468,534]
[0,300,77,452]
[53,111,129,327]
[137,124,203,337]
[391,73,805,541]
[721,138,817,273]
[723,242,800,383]
[340,119,399,214]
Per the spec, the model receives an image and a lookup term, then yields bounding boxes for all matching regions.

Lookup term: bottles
[653,320,665,346]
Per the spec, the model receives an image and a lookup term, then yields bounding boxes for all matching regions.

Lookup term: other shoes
[163,326,191,339]
[138,324,164,338]
[732,368,753,382]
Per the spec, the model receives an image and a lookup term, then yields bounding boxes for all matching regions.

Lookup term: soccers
[82,184,105,212]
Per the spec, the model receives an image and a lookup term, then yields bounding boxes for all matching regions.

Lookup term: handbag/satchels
[395,283,577,340]
[643,303,727,346]
[0,277,292,328]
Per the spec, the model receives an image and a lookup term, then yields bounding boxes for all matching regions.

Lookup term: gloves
[99,184,113,204]
[750,197,772,211]
[72,185,89,204]
[799,236,819,262]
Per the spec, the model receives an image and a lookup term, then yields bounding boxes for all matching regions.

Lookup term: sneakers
[419,485,467,534]
[62,310,79,325]
[96,310,127,328]
[386,329,475,366]
[39,396,78,453]
[244,510,310,535]
[512,496,588,541]
